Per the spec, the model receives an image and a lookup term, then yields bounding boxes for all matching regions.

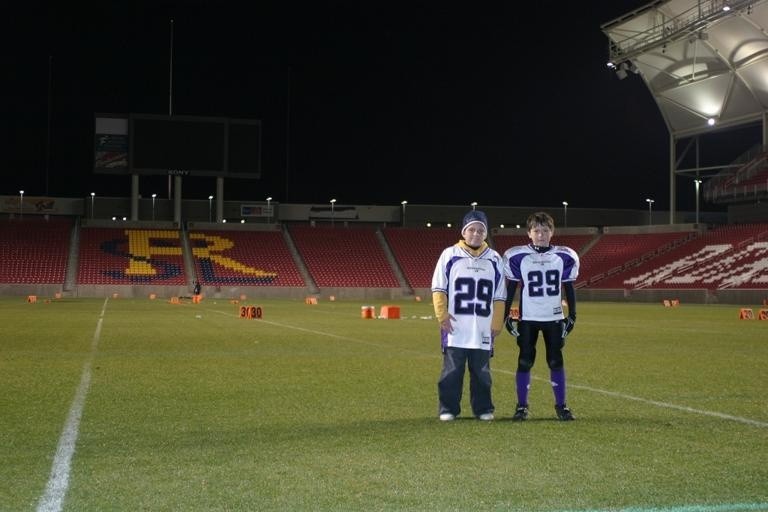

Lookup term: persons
[429,209,507,422]
[192,281,201,296]
[498,211,587,422]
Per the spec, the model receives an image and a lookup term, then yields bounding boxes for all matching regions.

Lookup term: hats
[461,210,489,240]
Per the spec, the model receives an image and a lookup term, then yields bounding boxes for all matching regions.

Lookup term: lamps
[723,0,730,11]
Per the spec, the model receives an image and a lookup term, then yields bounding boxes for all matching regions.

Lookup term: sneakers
[512,402,530,421]
[479,410,494,421]
[554,404,577,420]
[439,412,456,421]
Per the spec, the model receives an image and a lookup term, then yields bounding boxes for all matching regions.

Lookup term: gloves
[561,315,575,339]
[505,317,521,338]
